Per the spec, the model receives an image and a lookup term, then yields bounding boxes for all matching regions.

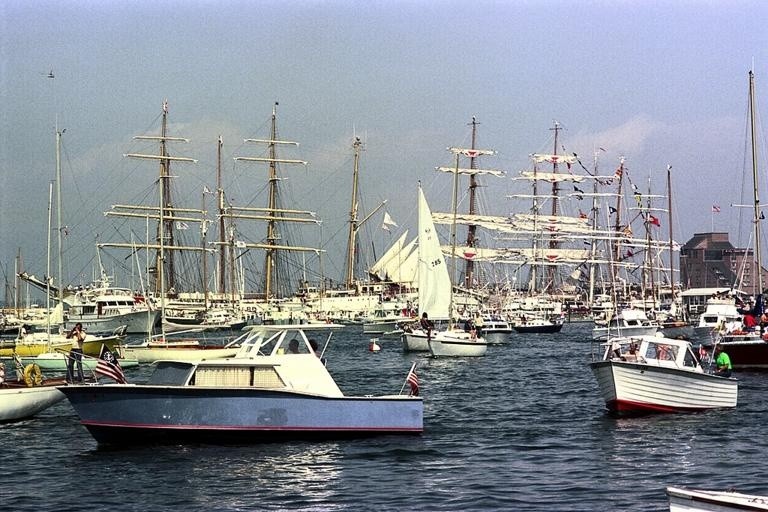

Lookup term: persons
[421,312,432,340]
[307,339,318,353]
[289,339,299,353]
[464,319,476,341]
[407,300,412,317]
[630,338,637,354]
[63,282,90,297]
[62,323,85,382]
[713,344,732,377]
[743,313,757,328]
[626,343,639,355]
[474,312,483,339]
[708,290,735,302]
[654,327,665,359]
[760,309,768,331]
[733,317,745,330]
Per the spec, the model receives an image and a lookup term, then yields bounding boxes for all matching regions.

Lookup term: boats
[54,325,423,443]
[669,486,768,512]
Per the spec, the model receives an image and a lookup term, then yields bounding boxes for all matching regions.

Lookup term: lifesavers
[24,363,41,385]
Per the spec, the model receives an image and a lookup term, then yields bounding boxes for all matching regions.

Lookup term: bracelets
[719,369,721,373]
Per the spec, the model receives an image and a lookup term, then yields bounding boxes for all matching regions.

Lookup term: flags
[649,215,659,227]
[408,366,419,396]
[712,205,721,214]
[95,344,125,384]
